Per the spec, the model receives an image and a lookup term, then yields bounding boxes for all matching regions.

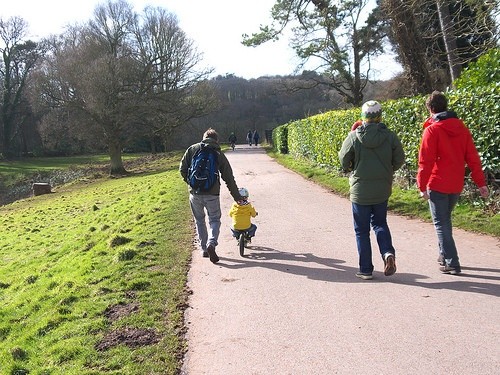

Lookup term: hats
[362,101,383,124]
[237,188,251,198]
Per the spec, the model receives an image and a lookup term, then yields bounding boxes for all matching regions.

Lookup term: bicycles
[231,212,258,256]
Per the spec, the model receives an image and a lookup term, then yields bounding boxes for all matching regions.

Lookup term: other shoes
[439,266,459,274]
[207,245,219,264]
[438,256,448,266]
[246,236,252,243]
[203,252,211,257]
[383,252,399,275]
[355,272,373,280]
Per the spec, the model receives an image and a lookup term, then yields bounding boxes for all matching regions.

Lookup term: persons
[229,129,259,147]
[229,188,258,242]
[180,128,243,263]
[338,100,404,280]
[417,92,490,272]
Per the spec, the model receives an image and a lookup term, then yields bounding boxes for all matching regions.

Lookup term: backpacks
[185,143,220,193]
